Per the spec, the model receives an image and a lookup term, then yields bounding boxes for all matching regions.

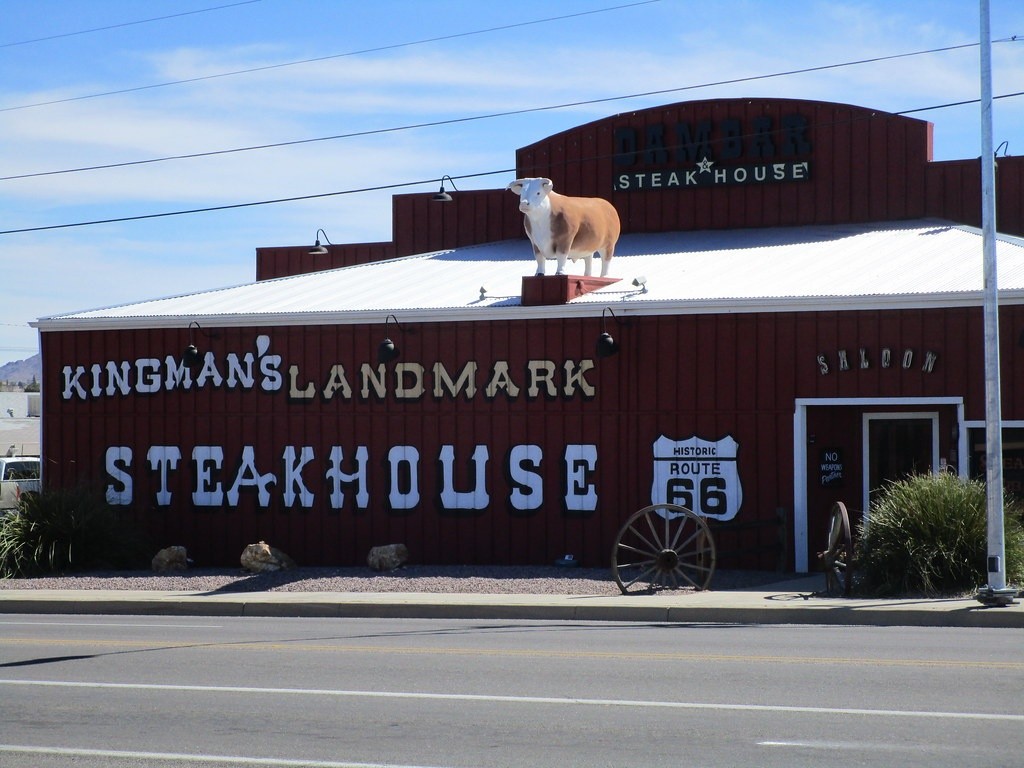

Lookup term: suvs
[0,455,41,511]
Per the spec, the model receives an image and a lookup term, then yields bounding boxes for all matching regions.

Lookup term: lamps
[977,141,1010,171]
[430,175,459,202]
[480,286,488,300]
[378,315,411,364]
[182,321,214,367]
[595,307,631,358]
[632,276,647,292]
[308,228,333,254]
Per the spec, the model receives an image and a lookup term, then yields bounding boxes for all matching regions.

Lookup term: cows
[504,176,621,277]
